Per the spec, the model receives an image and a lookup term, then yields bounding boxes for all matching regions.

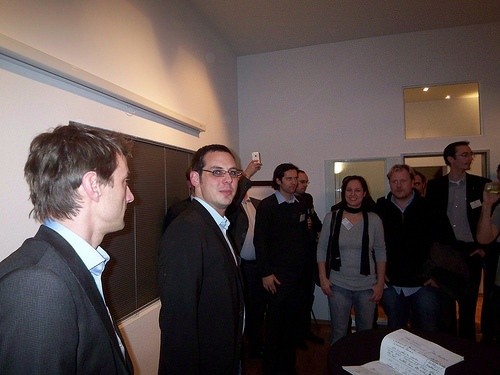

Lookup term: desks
[327,327,480,375]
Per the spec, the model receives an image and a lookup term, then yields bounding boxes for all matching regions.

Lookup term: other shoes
[299,341,310,351]
[308,334,324,344]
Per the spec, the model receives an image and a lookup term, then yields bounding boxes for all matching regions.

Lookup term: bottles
[307,209,312,229]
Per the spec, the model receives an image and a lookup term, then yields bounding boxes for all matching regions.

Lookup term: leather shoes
[251,345,264,357]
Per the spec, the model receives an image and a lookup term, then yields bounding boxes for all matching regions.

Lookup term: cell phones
[252,152,260,163]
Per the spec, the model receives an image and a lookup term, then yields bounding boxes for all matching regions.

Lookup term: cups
[485,182,500,193]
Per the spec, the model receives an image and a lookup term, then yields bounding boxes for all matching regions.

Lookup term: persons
[157,141,500,375]
[0,124,138,375]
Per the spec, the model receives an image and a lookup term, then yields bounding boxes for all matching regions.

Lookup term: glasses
[455,153,475,158]
[196,168,242,180]
